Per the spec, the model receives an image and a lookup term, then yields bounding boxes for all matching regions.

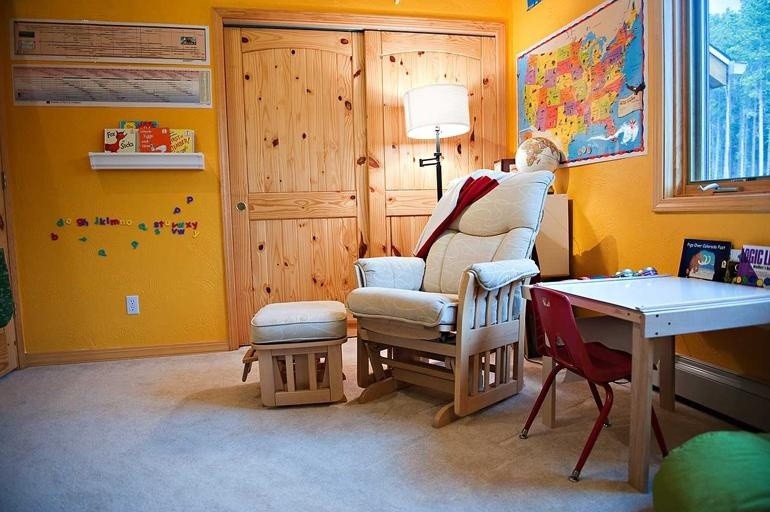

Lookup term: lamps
[402,83,471,203]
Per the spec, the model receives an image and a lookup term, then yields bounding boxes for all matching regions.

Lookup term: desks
[515,274,769,493]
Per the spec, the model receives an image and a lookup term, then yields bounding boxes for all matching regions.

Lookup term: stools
[242,300,347,406]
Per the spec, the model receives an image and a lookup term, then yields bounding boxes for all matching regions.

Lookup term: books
[723,247,741,283]
[167,128,195,153]
[734,243,770,291]
[138,119,159,129]
[118,119,142,130]
[138,126,172,153]
[676,238,733,282]
[102,128,139,153]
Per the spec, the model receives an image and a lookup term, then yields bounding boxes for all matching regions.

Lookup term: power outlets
[126,295,139,315]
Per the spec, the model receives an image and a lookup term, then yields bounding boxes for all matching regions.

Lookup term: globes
[515,137,561,193]
